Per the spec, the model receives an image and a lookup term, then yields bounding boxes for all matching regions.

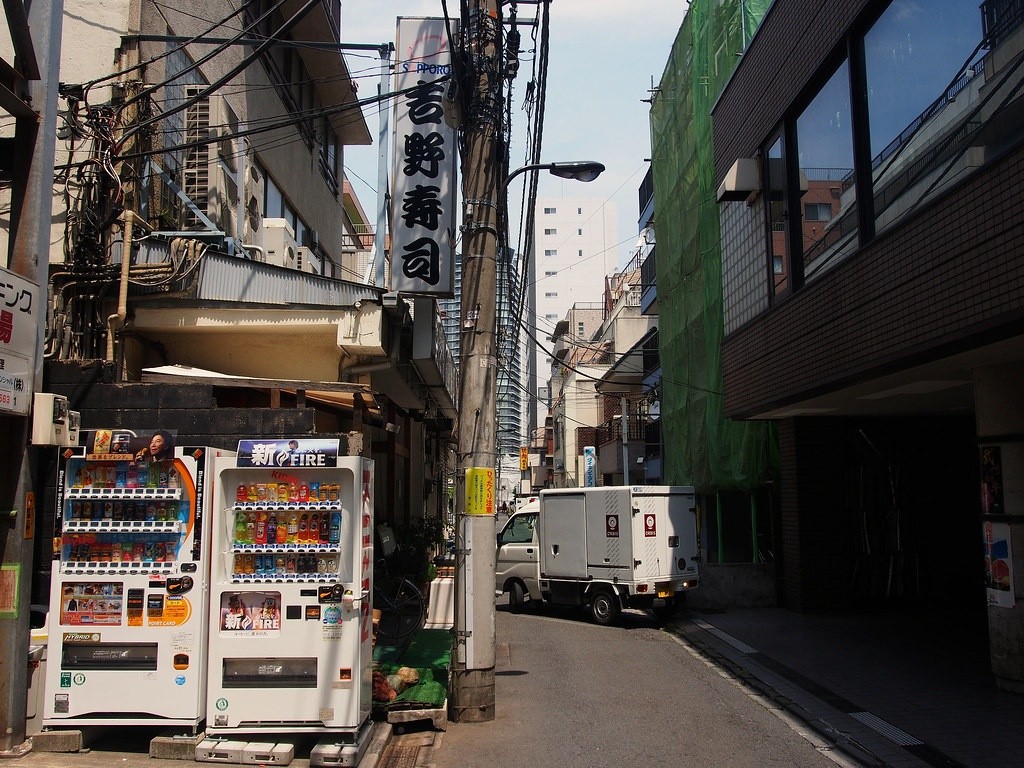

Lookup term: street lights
[445,1,611,724]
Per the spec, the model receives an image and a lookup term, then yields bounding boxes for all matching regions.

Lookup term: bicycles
[374,557,425,642]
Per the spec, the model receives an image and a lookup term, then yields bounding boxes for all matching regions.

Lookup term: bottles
[232,479,342,574]
[229,594,245,620]
[263,596,276,620]
[68,459,181,563]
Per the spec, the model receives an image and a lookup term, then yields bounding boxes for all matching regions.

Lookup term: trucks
[497,486,703,623]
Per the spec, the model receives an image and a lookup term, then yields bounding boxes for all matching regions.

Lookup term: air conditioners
[178,81,322,275]
